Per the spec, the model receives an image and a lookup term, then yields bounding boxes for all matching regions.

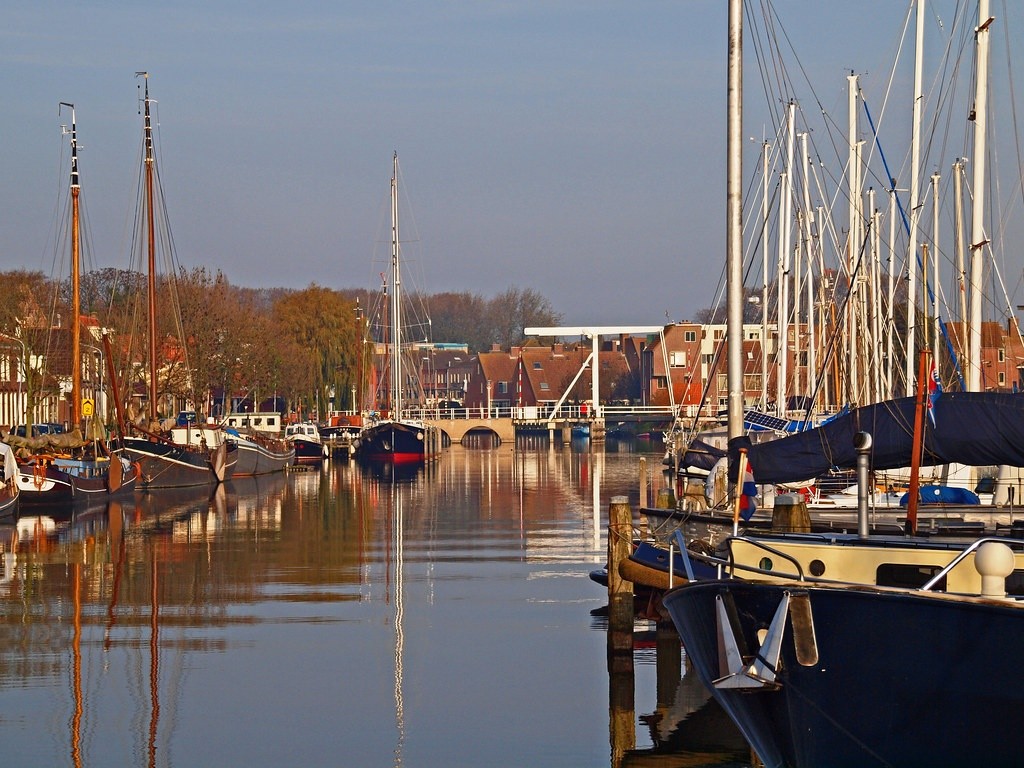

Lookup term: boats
[170,415,296,477]
[283,424,329,463]
[663,529,1024,768]
[0,443,20,517]
[617,430,1024,601]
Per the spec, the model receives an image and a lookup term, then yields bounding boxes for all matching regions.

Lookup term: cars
[34,424,56,435]
[10,424,41,438]
[175,412,197,425]
[46,424,66,434]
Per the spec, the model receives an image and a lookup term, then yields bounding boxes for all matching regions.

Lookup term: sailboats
[358,149,439,462]
[98,71,229,491]
[640,0,1024,530]
[16,101,141,506]
